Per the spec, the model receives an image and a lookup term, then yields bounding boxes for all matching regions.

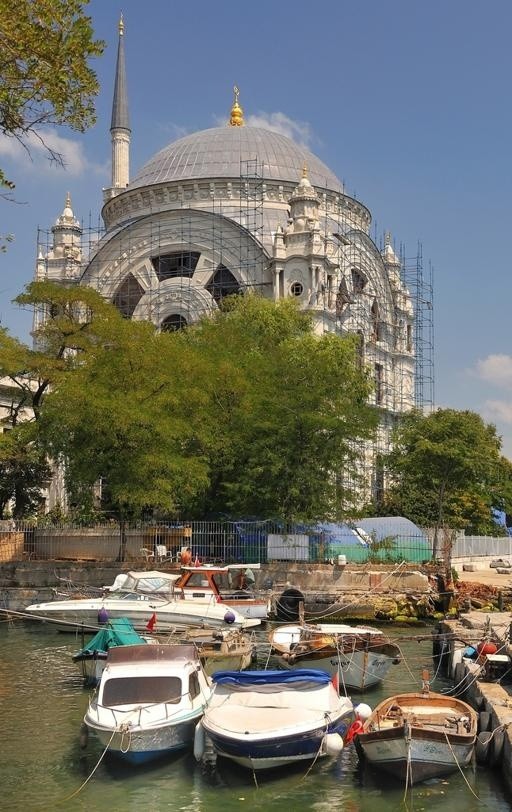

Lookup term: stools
[245,582,255,591]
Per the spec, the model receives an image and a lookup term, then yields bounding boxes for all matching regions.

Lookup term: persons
[234,567,256,590]
[180,546,192,568]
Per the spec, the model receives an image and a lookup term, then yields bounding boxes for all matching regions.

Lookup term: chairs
[140,548,154,562]
[176,547,189,563]
[157,546,172,563]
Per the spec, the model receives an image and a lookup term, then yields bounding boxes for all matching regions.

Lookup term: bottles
[194,552,200,566]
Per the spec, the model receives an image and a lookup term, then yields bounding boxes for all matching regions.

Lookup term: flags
[342,715,365,747]
[145,613,156,631]
[333,670,339,691]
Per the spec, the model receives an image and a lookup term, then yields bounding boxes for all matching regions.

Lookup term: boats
[71,636,160,686]
[84,645,212,763]
[25,570,245,633]
[358,670,478,786]
[177,622,257,675]
[55,566,270,617]
[203,669,354,769]
[267,601,400,692]
